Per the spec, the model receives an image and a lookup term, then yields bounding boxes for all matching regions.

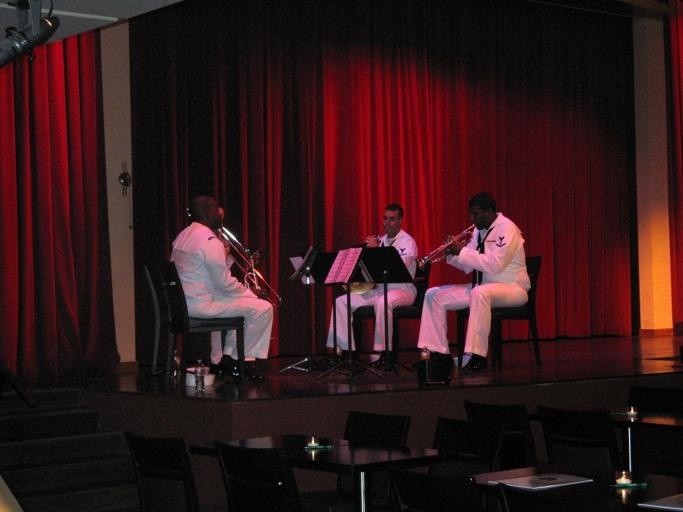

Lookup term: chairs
[353,259,430,366]
[142,264,170,377]
[454,254,540,372]
[161,261,246,385]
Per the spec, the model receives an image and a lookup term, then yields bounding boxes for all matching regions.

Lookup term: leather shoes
[208,355,485,383]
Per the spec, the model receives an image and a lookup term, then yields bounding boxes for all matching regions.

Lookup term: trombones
[219,226,280,305]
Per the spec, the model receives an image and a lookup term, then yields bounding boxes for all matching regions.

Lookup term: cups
[306,435,320,447]
[614,469,632,485]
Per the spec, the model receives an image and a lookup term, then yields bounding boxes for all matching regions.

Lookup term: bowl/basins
[185,374,214,387]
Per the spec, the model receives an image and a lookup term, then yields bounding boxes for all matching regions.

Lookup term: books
[322,247,361,283]
[469,464,594,494]
[290,246,315,285]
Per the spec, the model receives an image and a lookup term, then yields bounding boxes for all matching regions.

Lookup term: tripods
[302,285,347,369]
[353,283,414,378]
[315,285,385,393]
[277,266,329,377]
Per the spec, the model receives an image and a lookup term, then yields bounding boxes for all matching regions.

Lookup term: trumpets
[414,224,474,270]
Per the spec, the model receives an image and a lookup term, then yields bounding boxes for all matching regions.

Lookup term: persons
[413,190,532,378]
[326,201,418,365]
[166,192,273,384]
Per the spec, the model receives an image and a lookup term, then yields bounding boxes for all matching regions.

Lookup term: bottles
[171,349,181,384]
[193,359,205,392]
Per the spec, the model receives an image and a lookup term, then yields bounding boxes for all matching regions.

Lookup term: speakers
[417,360,450,386]
